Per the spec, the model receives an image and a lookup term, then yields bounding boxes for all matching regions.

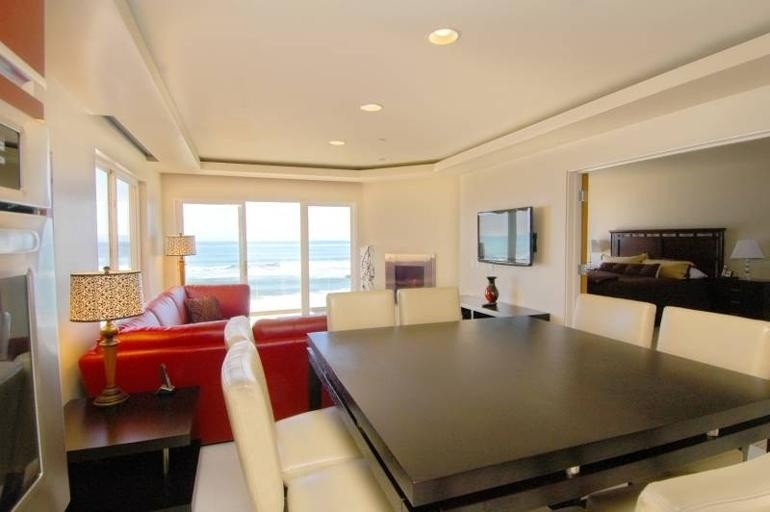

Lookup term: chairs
[222,312,365,478]
[568,289,657,351]
[653,303,770,379]
[398,285,463,327]
[220,342,407,511]
[325,287,397,329]
[590,440,770,511]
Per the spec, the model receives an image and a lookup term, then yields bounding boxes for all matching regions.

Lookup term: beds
[589,226,730,293]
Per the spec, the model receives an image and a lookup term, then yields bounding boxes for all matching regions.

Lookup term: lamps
[729,238,767,279]
[166,233,198,284]
[67,267,149,407]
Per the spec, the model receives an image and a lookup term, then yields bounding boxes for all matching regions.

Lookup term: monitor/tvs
[477,207,536,266]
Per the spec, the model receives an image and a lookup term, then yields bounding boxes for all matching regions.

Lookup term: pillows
[598,251,709,279]
[184,294,224,323]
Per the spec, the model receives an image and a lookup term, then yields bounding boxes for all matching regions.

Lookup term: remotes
[484,304,496,307]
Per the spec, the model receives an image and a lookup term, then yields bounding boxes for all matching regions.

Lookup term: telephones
[156,363,175,396]
[721,264,733,278]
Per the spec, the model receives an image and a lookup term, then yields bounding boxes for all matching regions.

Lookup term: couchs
[78,282,341,449]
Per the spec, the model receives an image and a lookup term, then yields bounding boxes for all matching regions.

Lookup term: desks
[304,312,770,511]
[459,294,551,324]
[60,386,201,508]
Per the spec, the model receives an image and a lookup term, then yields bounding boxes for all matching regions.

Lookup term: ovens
[0,96,72,512]
